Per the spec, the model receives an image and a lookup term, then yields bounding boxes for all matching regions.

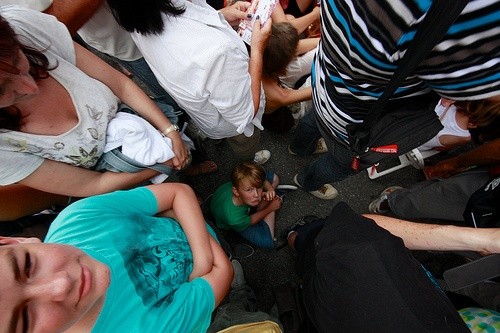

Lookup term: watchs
[162,125,179,137]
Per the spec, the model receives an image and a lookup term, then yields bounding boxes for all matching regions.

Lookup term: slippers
[183,160,217,178]
[190,184,209,206]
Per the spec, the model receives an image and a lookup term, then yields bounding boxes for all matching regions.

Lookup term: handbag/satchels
[273,280,304,333]
[346,90,444,171]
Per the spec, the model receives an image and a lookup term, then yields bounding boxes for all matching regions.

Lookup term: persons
[0,0,500,333]
[0,182,254,333]
[203,162,297,247]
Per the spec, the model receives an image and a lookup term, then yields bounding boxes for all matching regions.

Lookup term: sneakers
[262,185,297,201]
[225,239,254,260]
[368,186,404,214]
[294,173,338,199]
[231,260,246,288]
[280,215,319,242]
[292,100,308,119]
[253,149,271,164]
[288,138,329,155]
